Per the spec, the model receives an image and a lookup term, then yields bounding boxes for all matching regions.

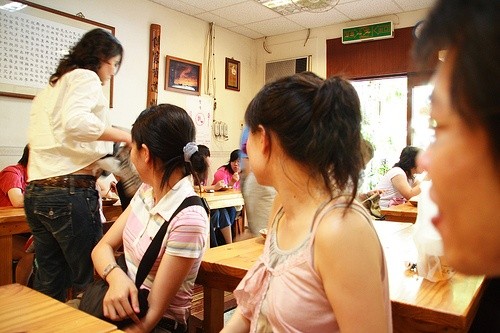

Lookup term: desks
[0,190,245,285]
[0,282,126,333]
[380,202,417,224]
[195,220,485,333]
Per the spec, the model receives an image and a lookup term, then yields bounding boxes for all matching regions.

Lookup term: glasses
[102,59,118,69]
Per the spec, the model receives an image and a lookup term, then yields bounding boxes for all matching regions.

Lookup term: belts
[32,176,96,189]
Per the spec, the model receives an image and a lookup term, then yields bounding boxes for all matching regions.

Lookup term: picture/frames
[165,55,202,96]
[225,57,241,92]
[0,0,115,108]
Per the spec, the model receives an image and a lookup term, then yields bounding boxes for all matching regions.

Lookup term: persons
[233,170,278,241]
[413,0,500,333]
[216,71,394,332]
[373,144,425,212]
[90,103,211,333]
[22,28,135,303]
[237,124,279,174]
[346,139,384,208]
[187,145,225,248]
[211,149,246,246]
[0,144,38,287]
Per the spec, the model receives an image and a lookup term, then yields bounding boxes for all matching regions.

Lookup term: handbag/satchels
[78,280,149,330]
[398,172,458,282]
[370,201,384,218]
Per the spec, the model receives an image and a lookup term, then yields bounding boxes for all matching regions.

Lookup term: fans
[290,0,340,13]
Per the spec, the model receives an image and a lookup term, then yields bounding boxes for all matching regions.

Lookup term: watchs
[99,262,121,280]
